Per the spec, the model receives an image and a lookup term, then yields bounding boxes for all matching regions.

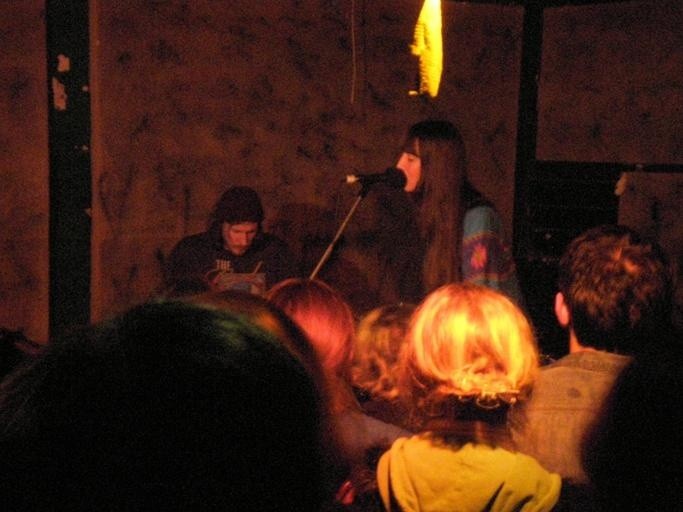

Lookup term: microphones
[347,169,408,193]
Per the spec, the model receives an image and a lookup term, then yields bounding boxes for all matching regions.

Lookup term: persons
[376,117,518,314]
[510,218,675,492]
[351,298,425,424]
[161,184,305,301]
[202,287,353,479]
[376,281,564,511]
[581,300,682,510]
[269,271,411,472]
[0,302,342,509]
